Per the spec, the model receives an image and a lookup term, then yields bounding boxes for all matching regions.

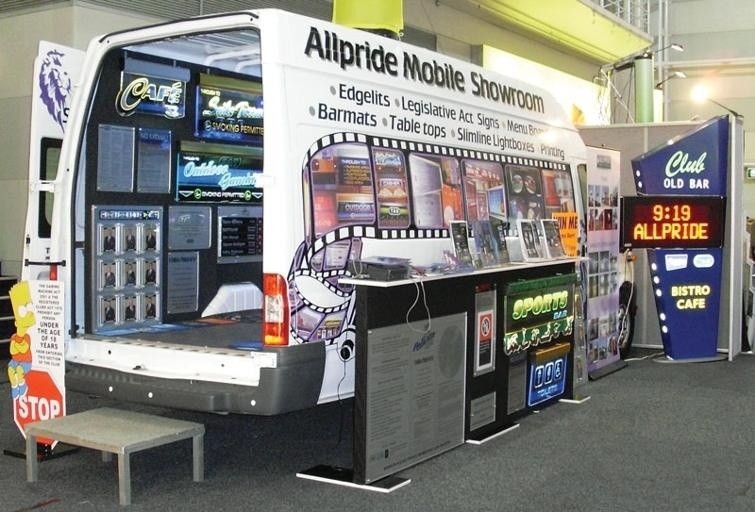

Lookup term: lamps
[656,71,686,87]
[653,42,684,54]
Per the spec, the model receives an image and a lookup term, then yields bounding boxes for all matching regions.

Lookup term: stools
[26,406,206,507]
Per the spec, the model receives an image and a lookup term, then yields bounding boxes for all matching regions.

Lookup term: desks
[295,250,592,494]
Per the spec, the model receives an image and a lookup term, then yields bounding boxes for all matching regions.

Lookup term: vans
[20,7,638,415]
